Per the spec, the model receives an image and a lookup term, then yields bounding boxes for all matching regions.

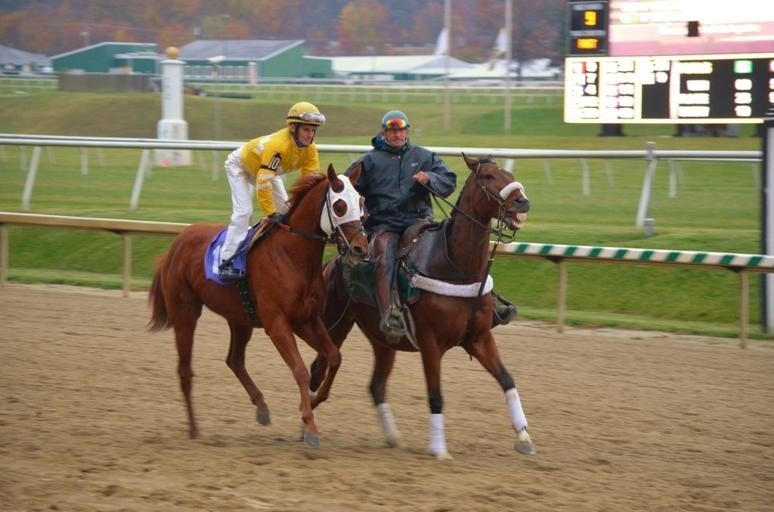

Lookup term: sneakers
[494,305,517,327]
[220,260,244,280]
[384,318,401,345]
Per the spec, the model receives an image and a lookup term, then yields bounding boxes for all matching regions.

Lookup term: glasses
[299,112,326,122]
[385,118,407,129]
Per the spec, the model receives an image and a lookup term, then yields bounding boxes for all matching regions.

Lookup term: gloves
[270,212,286,224]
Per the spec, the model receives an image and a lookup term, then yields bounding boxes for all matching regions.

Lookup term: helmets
[381,110,410,129]
[287,102,325,127]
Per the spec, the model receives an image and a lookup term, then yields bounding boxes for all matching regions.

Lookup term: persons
[219,101,325,276]
[342,110,517,344]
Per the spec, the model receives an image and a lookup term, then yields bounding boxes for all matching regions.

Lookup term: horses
[144,161,370,450]
[296,152,536,460]
